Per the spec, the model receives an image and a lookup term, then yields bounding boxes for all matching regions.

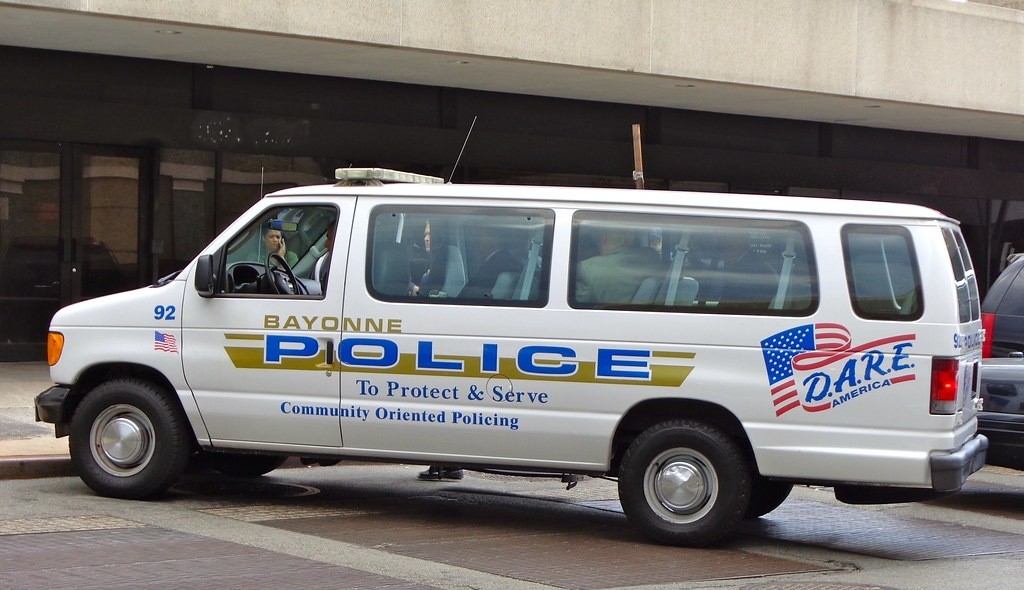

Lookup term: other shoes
[418,467,464,480]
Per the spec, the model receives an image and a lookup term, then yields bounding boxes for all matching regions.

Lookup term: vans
[33,168,988,548]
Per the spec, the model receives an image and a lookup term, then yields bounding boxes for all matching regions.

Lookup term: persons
[249,226,299,272]
[574,227,665,305]
[324,220,337,251]
[455,225,522,300]
[408,217,464,481]
[713,231,778,309]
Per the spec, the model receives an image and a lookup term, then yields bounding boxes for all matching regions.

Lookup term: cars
[981,253,1023,450]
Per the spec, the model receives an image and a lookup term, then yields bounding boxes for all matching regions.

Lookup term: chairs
[373,240,699,307]
